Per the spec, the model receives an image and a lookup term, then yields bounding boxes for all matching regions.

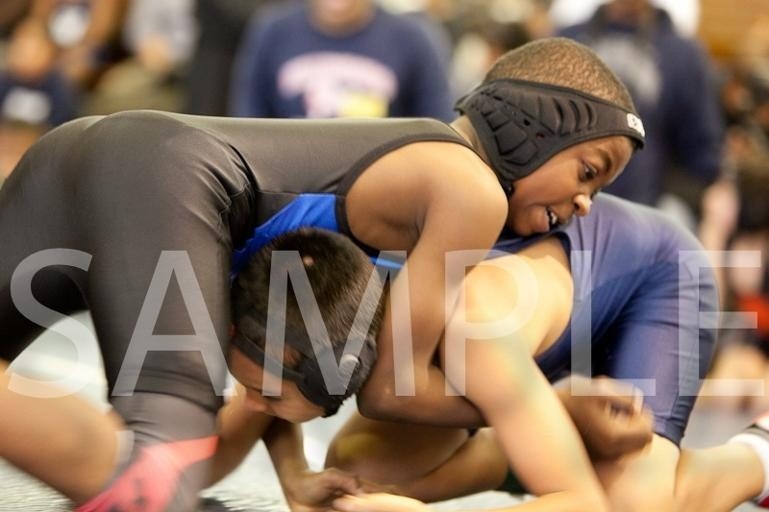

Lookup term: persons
[208,192,769,512]
[2,1,768,421]
[5,38,648,511]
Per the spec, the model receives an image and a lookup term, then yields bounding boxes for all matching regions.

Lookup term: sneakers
[64,436,225,512]
